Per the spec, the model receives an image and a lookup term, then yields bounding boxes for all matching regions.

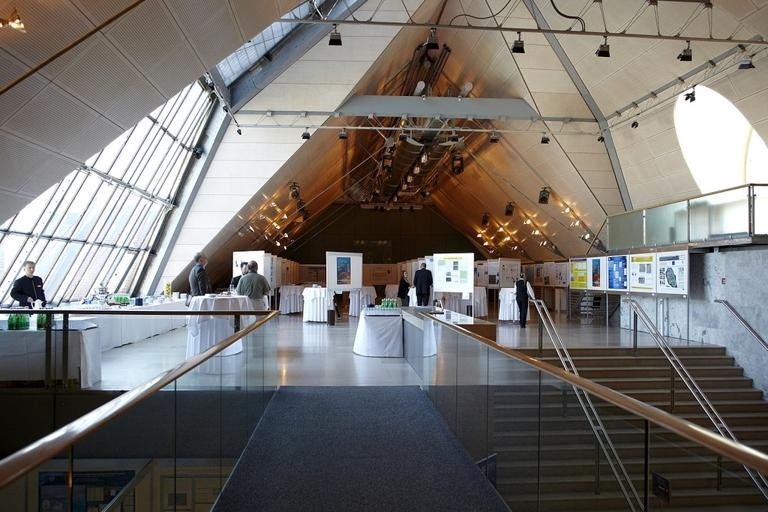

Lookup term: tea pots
[30,299,44,309]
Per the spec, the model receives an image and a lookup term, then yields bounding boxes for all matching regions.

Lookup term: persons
[188,256,212,296]
[413,262,433,306]
[230,262,248,333]
[236,260,271,322]
[397,269,412,306]
[512,273,536,328]
[9,261,47,317]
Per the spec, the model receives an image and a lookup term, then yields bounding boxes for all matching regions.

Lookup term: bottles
[113,293,130,306]
[37,307,48,330]
[7,306,30,330]
[381,297,398,308]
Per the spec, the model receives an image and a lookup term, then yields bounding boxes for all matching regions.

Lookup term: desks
[349,287,377,317]
[474,286,489,318]
[498,287,530,321]
[407,287,433,306]
[385,285,399,298]
[352,308,402,358]
